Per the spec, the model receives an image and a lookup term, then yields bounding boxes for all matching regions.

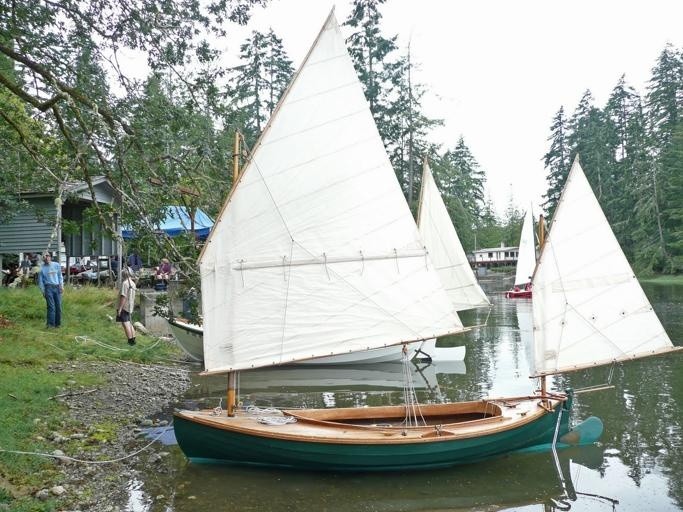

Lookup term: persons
[524,283,530,291]
[37,250,64,329]
[514,286,519,294]
[18,253,32,277]
[128,249,142,273]
[156,258,171,280]
[113,268,136,346]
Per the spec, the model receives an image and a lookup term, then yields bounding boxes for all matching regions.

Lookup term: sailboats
[505,198,544,300]
[170,4,681,479]
[163,155,493,378]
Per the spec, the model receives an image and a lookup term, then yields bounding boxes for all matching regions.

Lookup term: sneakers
[127,340,136,346]
[47,325,61,329]
